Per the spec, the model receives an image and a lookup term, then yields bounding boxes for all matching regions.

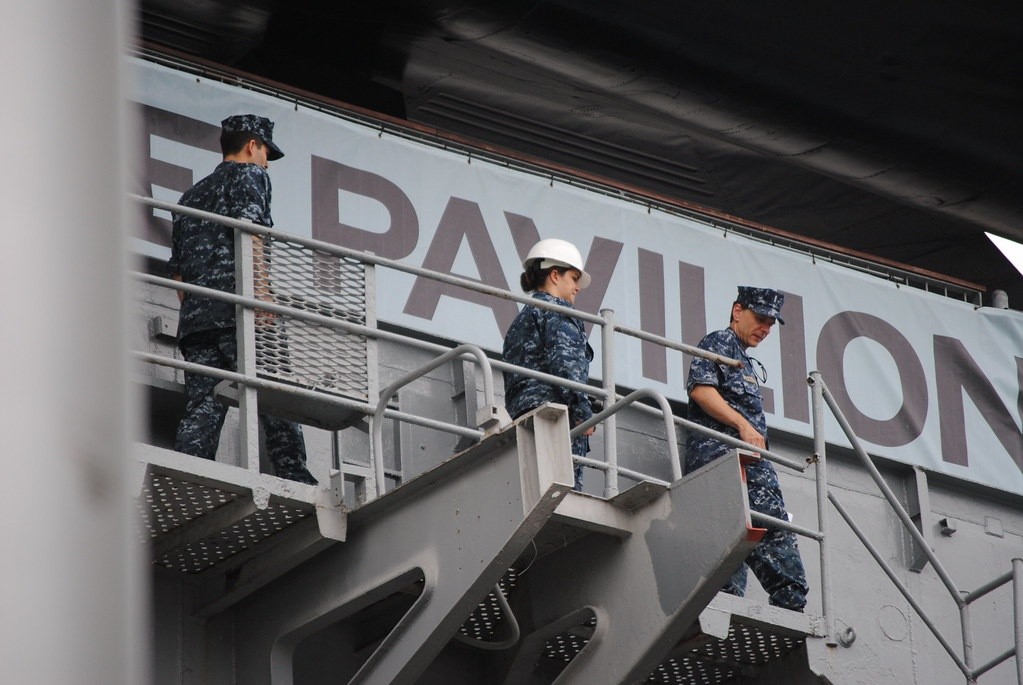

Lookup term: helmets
[521,239,591,289]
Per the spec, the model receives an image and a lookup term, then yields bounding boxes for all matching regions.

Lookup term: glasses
[746,354,768,383]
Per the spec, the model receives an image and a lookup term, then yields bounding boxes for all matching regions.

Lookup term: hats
[736,285,787,325]
[221,114,285,161]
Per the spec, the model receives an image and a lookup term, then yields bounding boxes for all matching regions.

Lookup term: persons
[504,239,596,491]
[170,115,318,484]
[688,286,811,613]
